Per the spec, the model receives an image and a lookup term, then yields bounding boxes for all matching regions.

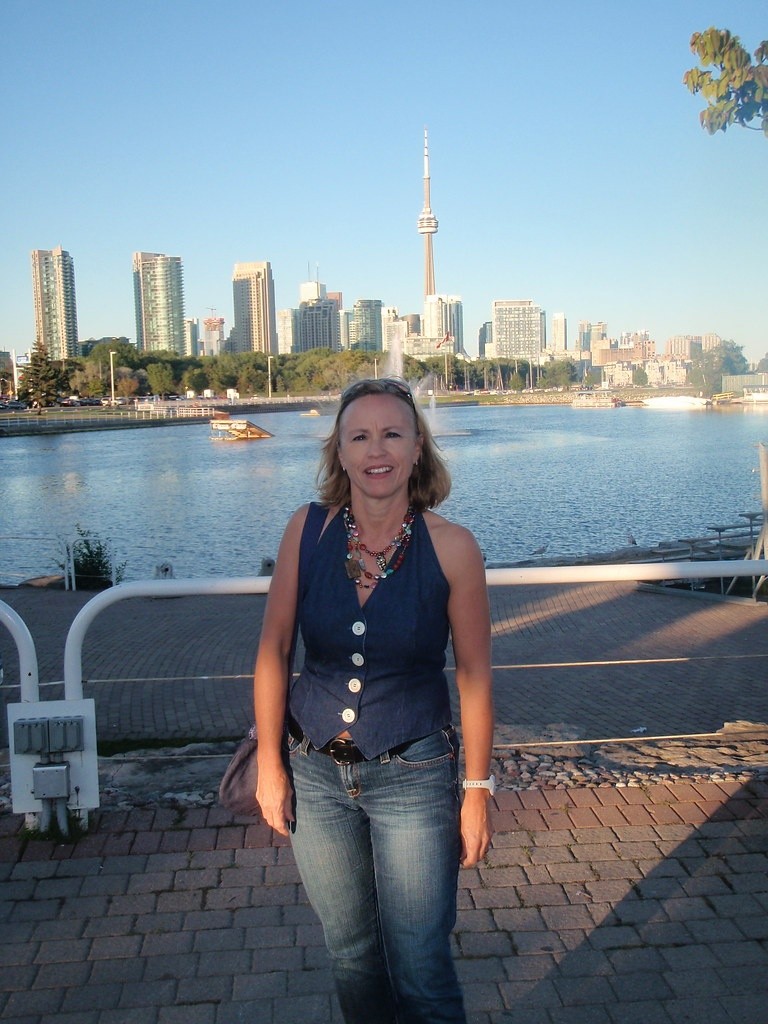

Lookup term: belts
[289,716,369,765]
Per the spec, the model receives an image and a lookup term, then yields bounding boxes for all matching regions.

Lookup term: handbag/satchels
[218,725,260,817]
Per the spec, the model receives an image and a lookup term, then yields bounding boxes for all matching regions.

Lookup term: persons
[253,377,493,1024]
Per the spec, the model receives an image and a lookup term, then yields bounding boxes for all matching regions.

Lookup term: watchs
[462,775,496,796]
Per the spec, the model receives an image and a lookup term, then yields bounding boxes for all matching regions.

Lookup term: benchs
[630,511,764,604]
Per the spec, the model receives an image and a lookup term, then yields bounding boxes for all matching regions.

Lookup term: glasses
[352,378,419,434]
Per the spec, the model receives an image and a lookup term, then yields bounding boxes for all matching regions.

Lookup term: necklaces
[343,503,416,590]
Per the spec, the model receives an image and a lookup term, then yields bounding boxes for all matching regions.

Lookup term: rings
[485,849,489,852]
